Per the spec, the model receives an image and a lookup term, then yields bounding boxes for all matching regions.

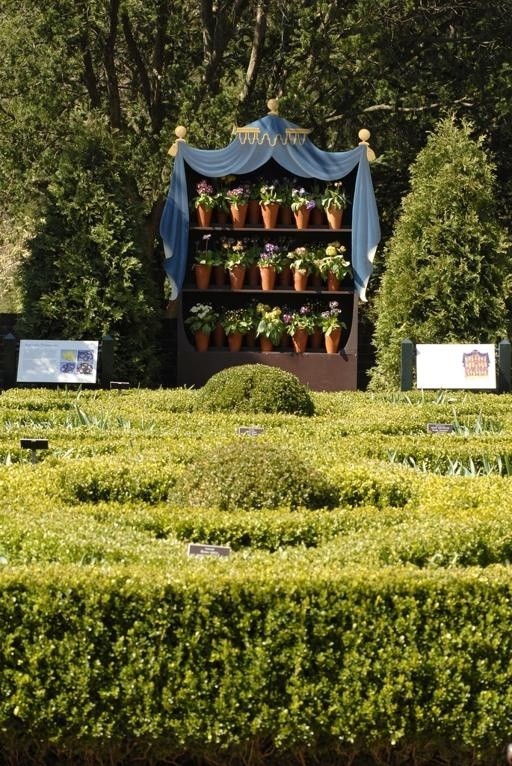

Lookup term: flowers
[184,180,353,346]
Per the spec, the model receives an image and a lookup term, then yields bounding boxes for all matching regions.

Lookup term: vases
[194,328,341,354]
